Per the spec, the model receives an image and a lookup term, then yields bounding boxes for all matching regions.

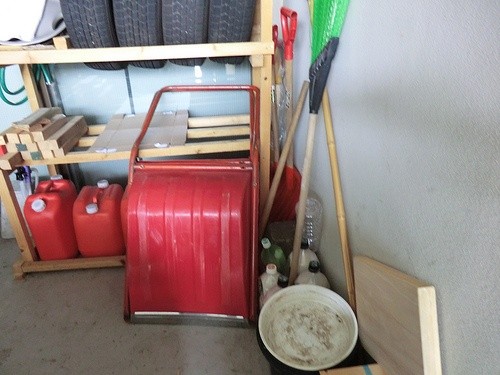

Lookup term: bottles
[261,264,281,290]
[260,275,289,309]
[296,197,322,251]
[295,261,330,288]
[287,239,319,282]
[260,237,288,275]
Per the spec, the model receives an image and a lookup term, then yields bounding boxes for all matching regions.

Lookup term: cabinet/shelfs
[0,0,275,279]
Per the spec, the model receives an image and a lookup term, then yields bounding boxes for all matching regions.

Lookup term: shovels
[270,7,301,218]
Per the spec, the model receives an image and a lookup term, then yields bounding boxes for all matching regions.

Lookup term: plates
[258,284,358,370]
